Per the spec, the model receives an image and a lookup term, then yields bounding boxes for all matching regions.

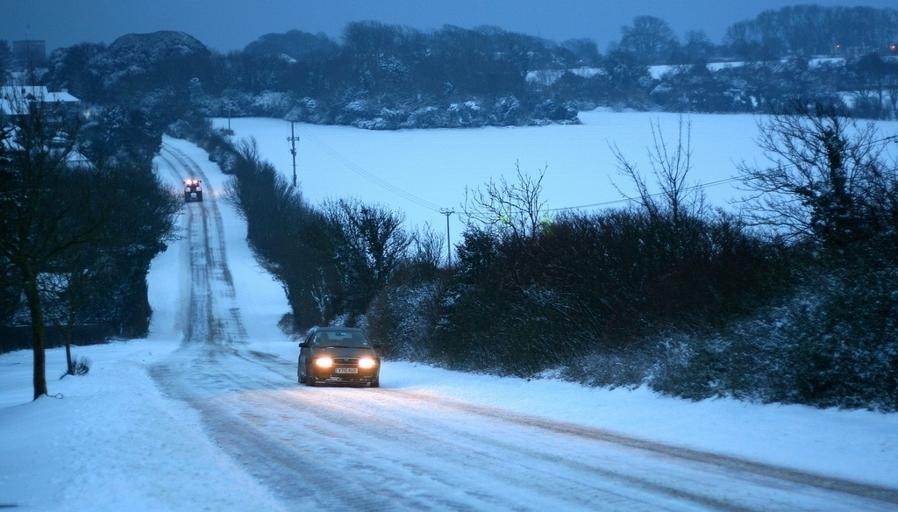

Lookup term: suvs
[182,180,204,202]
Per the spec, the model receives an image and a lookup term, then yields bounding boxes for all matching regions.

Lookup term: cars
[298,325,380,389]
